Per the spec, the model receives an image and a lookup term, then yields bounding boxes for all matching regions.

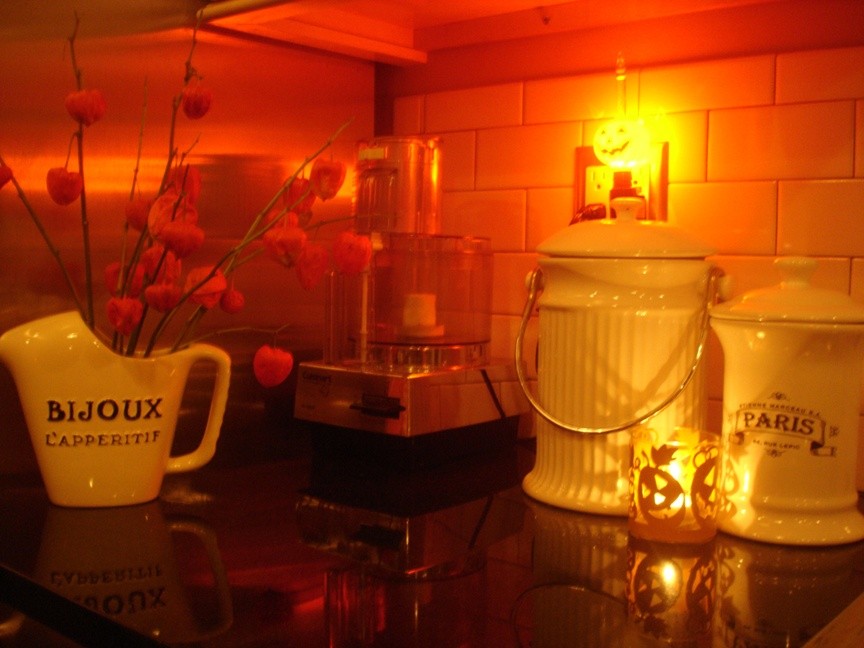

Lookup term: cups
[631,423,723,546]
[513,197,714,517]
[707,280,864,545]
[323,132,492,376]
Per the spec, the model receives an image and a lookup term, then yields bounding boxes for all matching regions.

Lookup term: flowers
[0,6,376,388]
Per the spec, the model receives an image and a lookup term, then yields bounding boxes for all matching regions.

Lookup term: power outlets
[585,164,649,218]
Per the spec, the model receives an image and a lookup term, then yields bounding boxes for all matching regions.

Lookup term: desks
[0,423,864,648]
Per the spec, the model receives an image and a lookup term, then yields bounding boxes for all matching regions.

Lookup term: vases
[0,312,231,509]
